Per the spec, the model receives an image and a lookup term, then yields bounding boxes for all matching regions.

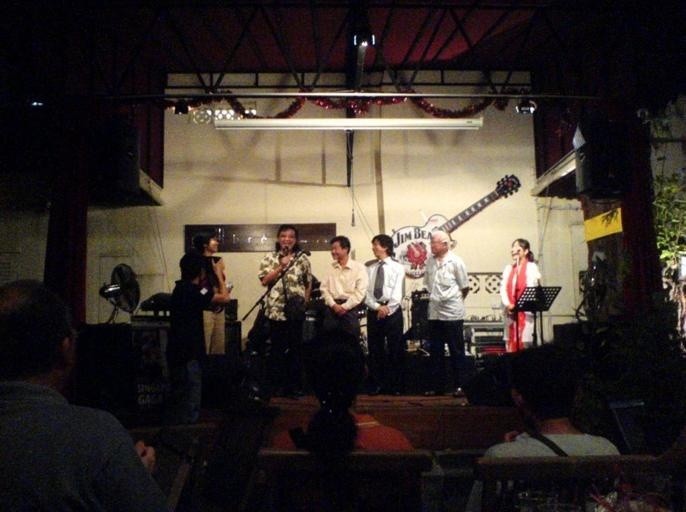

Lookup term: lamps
[515,98,536,114]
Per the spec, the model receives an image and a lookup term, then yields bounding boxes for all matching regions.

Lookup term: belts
[377,299,386,305]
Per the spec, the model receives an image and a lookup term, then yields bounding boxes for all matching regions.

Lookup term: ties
[374,260,385,301]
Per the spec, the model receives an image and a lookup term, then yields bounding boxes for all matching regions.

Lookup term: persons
[423,230,471,398]
[258,227,313,397]
[483,346,620,457]
[502,238,541,352]
[0,225,623,512]
[1,279,173,512]
[166,228,230,425]
[318,234,408,392]
[270,345,411,479]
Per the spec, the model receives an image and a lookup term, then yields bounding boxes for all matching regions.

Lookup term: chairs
[465,457,674,512]
[260,451,487,511]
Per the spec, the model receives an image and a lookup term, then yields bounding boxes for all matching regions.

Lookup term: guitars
[390,173,521,279]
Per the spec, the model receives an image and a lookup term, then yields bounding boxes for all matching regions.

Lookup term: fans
[99,263,140,324]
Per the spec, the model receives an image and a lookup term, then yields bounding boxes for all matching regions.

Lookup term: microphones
[512,249,520,266]
[283,247,289,255]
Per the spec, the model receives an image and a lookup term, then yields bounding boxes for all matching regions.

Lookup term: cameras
[202,256,225,288]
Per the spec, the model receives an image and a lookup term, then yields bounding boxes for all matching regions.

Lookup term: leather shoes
[367,384,465,400]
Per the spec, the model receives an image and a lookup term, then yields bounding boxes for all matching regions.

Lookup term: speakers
[90,120,139,203]
[463,327,507,361]
[204,299,241,355]
[79,324,169,428]
[574,123,631,200]
[553,322,588,342]
